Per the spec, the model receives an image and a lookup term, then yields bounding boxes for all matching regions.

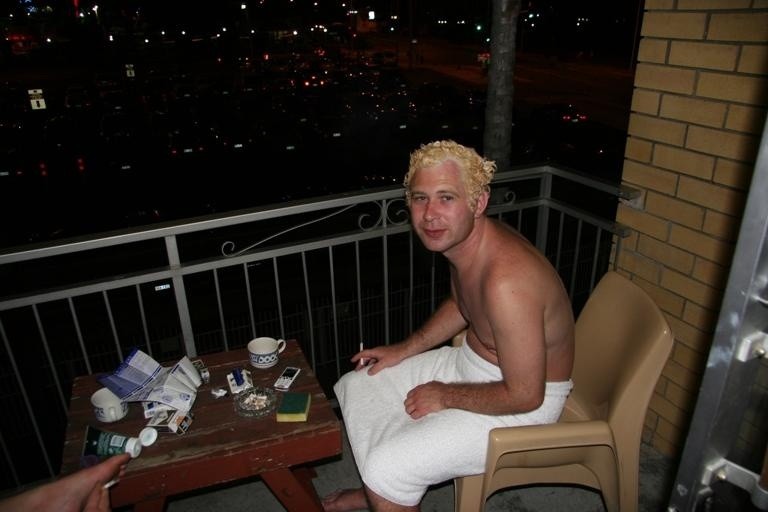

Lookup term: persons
[0,452,131,512]
[319,139,575,512]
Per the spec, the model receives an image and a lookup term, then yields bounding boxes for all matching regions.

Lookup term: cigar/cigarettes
[360,342,364,365]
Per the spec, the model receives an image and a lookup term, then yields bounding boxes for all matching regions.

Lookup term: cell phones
[273,366,301,392]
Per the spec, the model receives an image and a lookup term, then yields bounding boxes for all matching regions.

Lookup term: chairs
[452,270,675,511]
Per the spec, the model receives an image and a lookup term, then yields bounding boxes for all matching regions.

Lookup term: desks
[60,339,345,511]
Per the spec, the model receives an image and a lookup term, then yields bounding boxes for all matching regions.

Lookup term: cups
[247,336,287,370]
[90,387,129,424]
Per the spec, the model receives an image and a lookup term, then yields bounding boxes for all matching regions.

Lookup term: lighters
[232,368,245,386]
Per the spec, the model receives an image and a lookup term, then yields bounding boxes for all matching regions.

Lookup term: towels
[332,337,574,508]
